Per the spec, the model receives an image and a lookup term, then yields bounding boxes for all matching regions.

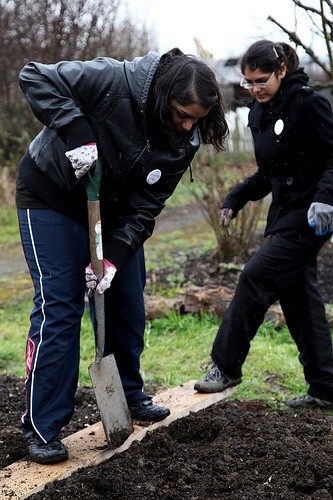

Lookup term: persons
[193,40,333,406]
[14,47,229,464]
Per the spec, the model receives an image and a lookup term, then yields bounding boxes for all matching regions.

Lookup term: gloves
[65,142,98,179]
[85,258,117,297]
[307,202,333,235]
[219,208,234,240]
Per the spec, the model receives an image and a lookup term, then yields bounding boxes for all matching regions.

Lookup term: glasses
[239,71,274,90]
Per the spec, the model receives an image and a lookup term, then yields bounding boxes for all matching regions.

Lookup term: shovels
[83,154,134,450]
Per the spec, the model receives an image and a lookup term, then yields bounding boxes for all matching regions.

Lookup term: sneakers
[286,394,333,410]
[194,361,242,393]
[23,427,68,464]
[125,399,170,422]
